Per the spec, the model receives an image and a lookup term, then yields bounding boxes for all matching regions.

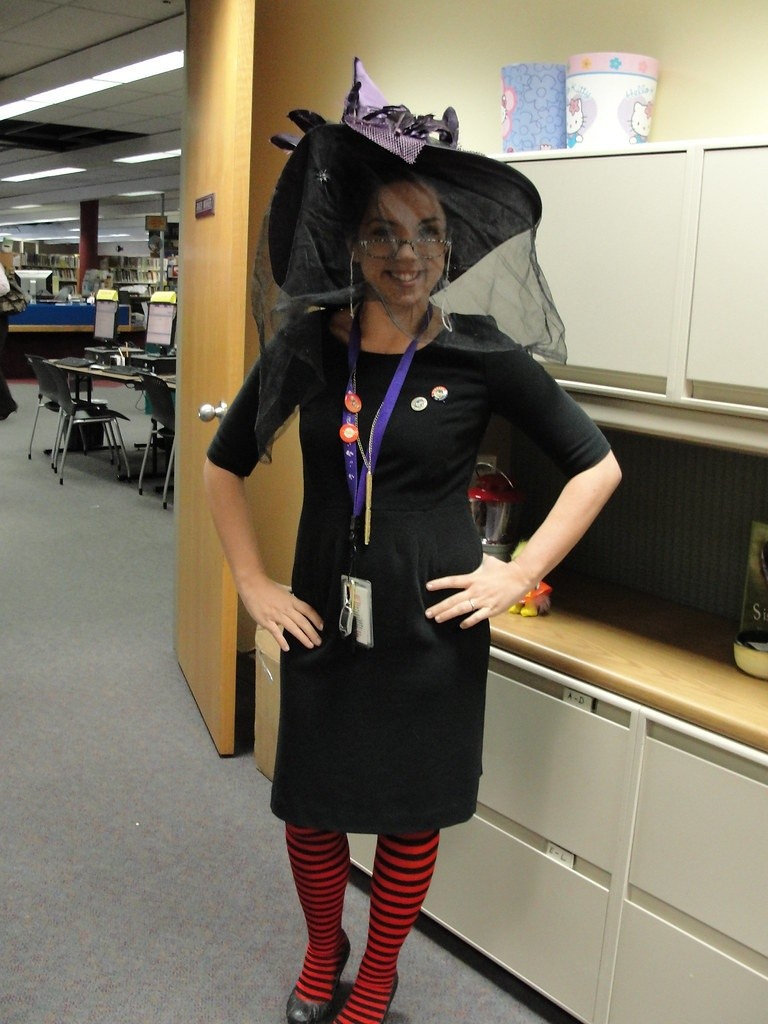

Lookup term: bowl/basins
[733,642,768,679]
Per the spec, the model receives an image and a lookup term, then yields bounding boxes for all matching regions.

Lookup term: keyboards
[101,364,148,375]
[53,356,96,367]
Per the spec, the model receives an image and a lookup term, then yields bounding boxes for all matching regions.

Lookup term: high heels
[286,928,351,1024]
[332,972,399,1024]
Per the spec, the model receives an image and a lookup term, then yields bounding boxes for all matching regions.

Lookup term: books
[15,253,168,294]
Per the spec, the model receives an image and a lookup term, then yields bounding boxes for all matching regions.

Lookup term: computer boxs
[84,345,144,365]
[129,354,176,374]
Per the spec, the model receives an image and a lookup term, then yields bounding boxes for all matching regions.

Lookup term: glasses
[351,225,454,261]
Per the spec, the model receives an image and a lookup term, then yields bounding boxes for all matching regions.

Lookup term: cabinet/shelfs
[347,569,768,1024]
[19,252,178,297]
[489,136,768,459]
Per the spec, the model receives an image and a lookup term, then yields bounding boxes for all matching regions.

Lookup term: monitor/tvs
[92,297,119,351]
[143,302,177,358]
[13,269,52,304]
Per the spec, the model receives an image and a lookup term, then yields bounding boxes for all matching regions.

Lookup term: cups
[116,356,125,367]
[87,299,94,305]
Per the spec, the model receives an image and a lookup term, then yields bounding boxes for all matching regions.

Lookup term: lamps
[0,50,185,183]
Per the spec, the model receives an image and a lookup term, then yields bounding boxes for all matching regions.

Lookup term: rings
[469,600,475,611]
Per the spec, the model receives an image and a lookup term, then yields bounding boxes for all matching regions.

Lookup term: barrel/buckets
[468,462,515,545]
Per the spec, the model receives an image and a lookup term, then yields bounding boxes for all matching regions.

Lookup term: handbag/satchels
[0,265,32,315]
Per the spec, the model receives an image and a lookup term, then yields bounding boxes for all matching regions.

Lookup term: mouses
[88,363,104,370]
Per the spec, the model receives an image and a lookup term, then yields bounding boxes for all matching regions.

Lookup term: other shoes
[0,399,17,420]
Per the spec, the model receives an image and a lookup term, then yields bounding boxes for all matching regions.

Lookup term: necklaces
[352,370,382,545]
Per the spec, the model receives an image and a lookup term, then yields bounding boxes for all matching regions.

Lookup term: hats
[267,57,541,307]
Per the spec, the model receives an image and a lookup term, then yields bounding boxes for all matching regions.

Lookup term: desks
[44,359,175,474]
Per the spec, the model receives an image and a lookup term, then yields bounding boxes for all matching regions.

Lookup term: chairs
[137,372,175,510]
[26,355,133,486]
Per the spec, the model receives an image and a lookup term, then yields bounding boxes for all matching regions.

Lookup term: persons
[0,263,18,420]
[204,125,622,1024]
